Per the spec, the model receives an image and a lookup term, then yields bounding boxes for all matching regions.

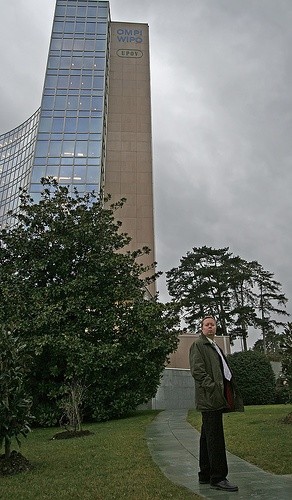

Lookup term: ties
[212,342,232,381]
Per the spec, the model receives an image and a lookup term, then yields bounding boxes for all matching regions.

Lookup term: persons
[189,316,246,492]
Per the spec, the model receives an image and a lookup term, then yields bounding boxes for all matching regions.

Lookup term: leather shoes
[199,478,239,492]
